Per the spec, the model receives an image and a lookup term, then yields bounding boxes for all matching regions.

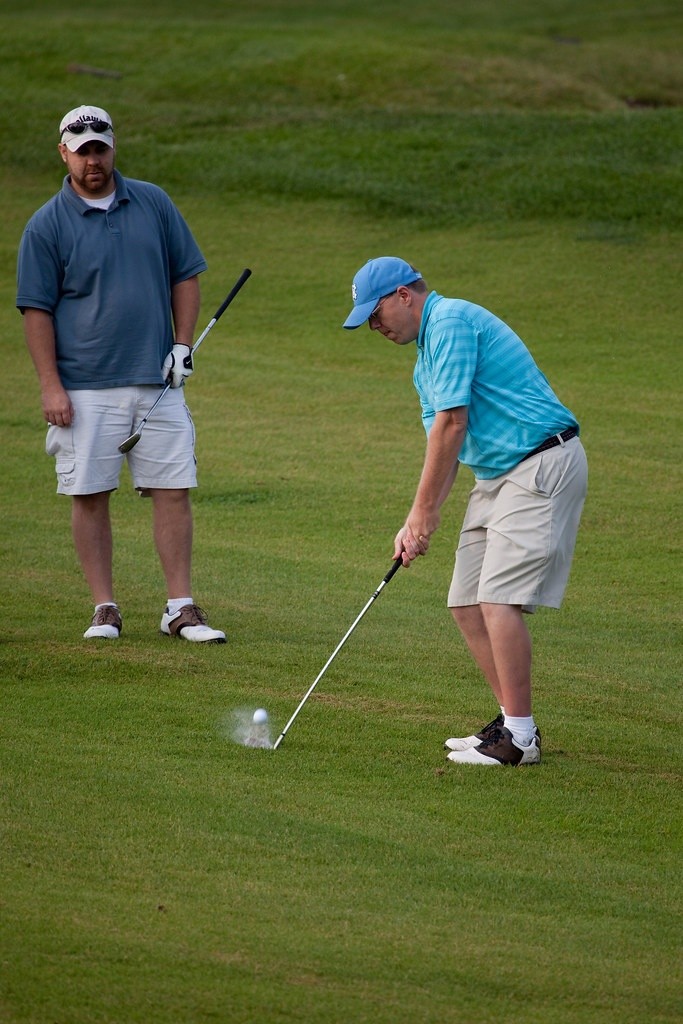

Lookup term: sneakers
[160,604,225,644]
[82,605,122,639]
[444,715,543,766]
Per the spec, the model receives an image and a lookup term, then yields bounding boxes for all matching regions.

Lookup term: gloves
[161,343,195,389]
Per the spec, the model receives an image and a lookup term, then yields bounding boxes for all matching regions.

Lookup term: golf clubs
[117,267,252,454]
[244,548,406,751]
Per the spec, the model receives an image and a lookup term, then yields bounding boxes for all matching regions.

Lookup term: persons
[340,255,587,765]
[17,104,227,644]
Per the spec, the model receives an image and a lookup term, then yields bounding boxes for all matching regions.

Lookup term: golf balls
[252,707,269,726]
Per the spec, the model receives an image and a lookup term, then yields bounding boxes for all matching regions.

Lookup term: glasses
[59,121,114,142]
[368,293,392,320]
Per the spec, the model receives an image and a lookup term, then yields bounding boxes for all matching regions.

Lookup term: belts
[520,429,575,461]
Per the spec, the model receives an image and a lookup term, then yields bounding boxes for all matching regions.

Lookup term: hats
[59,104,114,153]
[343,257,422,330]
